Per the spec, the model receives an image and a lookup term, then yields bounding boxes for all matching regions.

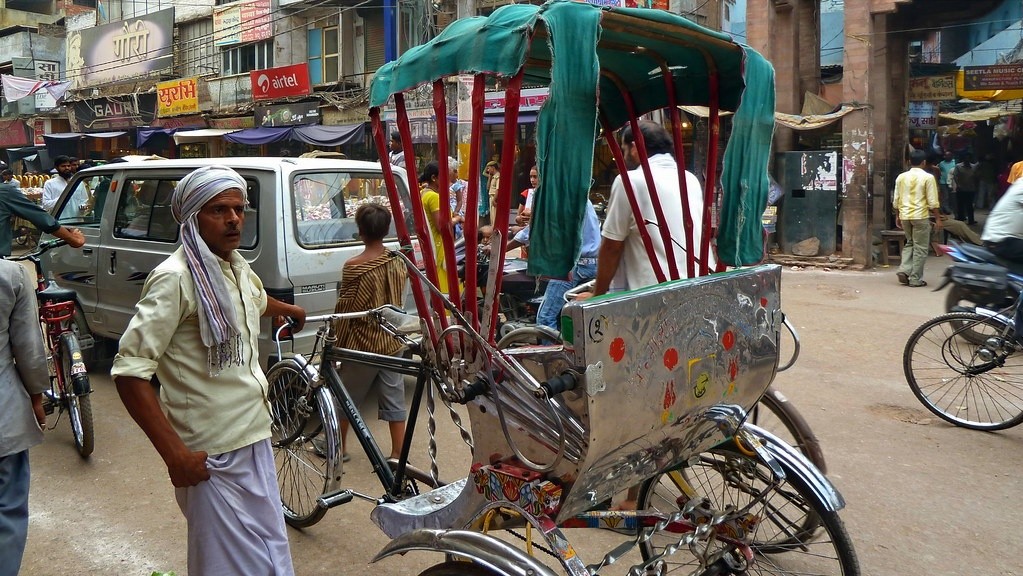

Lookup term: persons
[65,30,92,90]
[0,260,50,576]
[262,109,274,127]
[111,165,307,576]
[388,120,712,345]
[892,115,1023,287]
[0,155,174,260]
[314,204,410,473]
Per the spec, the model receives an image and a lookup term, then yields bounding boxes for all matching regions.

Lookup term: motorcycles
[930,238,1023,347]
[455,229,550,349]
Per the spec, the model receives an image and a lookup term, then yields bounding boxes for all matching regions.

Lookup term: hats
[487,161,497,165]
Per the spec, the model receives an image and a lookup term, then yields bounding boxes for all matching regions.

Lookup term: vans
[35,156,432,375]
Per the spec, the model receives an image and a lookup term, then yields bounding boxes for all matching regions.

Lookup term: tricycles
[267,264,864,576]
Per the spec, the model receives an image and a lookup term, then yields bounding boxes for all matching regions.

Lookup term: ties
[488,176,494,189]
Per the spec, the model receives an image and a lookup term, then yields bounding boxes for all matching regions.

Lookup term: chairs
[129,182,173,236]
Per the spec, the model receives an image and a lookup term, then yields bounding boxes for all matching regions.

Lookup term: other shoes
[898,272,909,283]
[909,280,927,287]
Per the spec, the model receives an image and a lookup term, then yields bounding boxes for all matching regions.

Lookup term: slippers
[313,433,351,461]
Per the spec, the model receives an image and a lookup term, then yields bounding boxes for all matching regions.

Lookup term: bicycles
[903,263,1023,431]
[5,236,94,458]
[665,387,829,554]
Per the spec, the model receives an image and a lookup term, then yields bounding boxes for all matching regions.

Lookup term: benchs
[497,259,782,480]
[296,219,359,244]
[881,229,907,266]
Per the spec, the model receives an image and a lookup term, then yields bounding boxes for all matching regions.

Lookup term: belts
[577,257,597,265]
